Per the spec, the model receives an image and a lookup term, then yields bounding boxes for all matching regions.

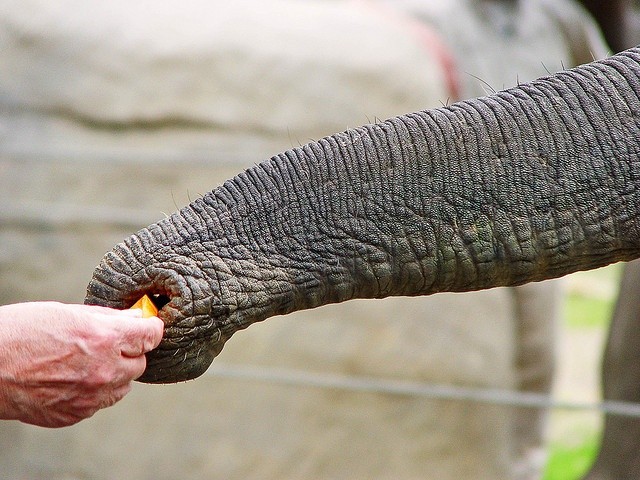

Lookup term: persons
[0,301,164,428]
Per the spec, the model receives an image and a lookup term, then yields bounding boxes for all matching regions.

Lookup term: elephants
[85,45,640,382]
